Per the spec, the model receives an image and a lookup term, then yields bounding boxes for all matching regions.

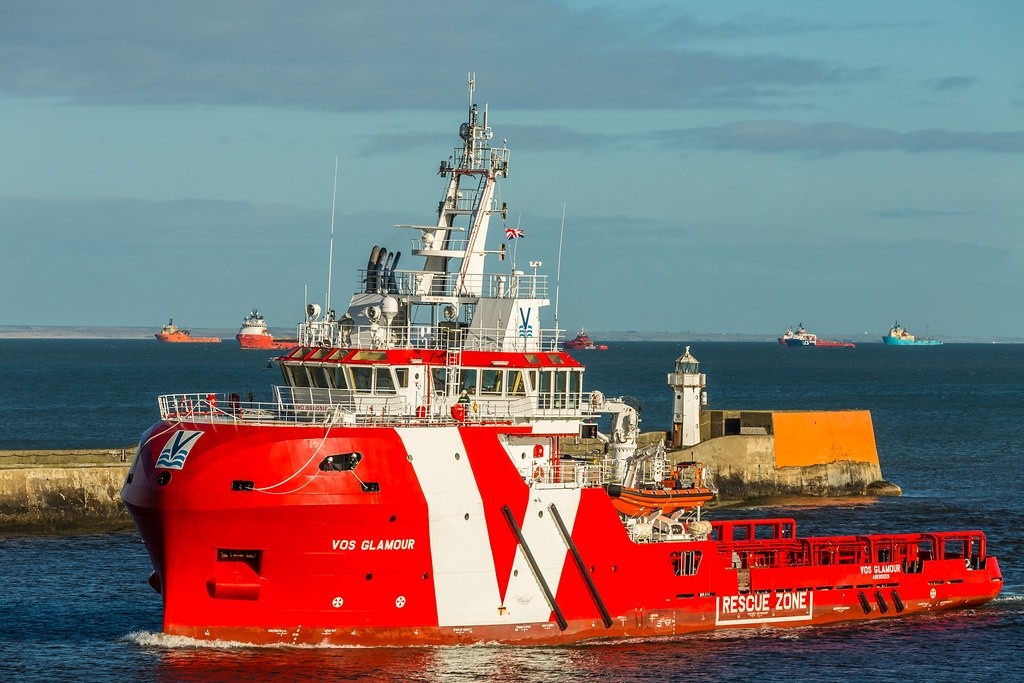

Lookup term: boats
[600,460,717,520]
[235,309,299,351]
[777,322,856,348]
[561,327,608,350]
[155,316,222,343]
[882,319,944,346]
[121,70,1006,650]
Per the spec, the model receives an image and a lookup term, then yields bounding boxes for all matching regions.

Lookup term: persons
[458,390,471,426]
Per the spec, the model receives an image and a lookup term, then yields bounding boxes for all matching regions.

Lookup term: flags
[506,228,525,240]
